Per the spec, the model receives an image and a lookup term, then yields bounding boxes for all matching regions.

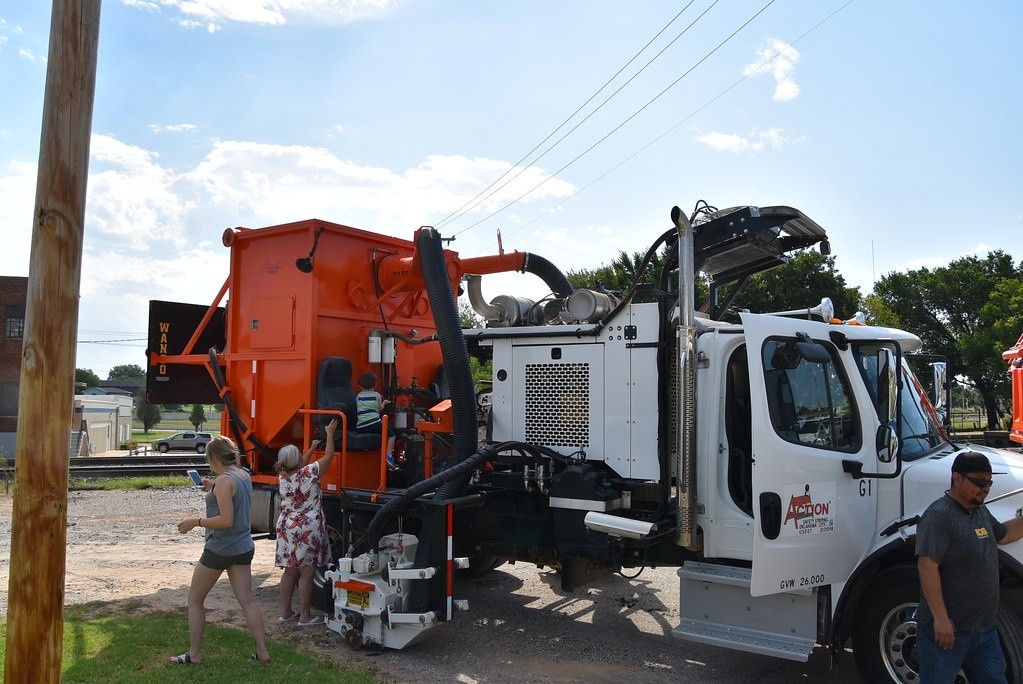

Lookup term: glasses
[957,473,993,488]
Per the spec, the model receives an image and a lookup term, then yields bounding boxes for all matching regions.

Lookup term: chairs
[312,357,382,452]
[428,363,451,403]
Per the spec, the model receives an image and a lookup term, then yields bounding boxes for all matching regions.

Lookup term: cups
[339,557,352,582]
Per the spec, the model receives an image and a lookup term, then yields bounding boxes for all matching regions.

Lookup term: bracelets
[199,518,201,526]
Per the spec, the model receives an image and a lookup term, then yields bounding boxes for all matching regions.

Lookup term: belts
[954,623,994,635]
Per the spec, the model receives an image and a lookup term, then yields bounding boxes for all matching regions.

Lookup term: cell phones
[187,470,205,488]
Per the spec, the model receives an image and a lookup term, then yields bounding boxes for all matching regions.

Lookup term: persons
[274,418,338,625]
[168,436,271,663]
[914,451,1023,684]
[356,372,397,469]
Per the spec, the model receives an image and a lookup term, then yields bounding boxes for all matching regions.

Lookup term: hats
[951,451,992,472]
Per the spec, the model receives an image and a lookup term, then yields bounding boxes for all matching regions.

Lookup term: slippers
[250,651,271,664]
[279,610,300,622]
[167,652,205,664]
[298,615,324,627]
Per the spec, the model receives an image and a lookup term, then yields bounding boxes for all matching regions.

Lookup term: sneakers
[386,453,396,469]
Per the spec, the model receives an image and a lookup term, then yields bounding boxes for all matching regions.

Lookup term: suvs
[152,432,213,454]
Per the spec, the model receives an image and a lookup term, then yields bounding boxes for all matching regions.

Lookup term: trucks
[146,198,1023,684]
